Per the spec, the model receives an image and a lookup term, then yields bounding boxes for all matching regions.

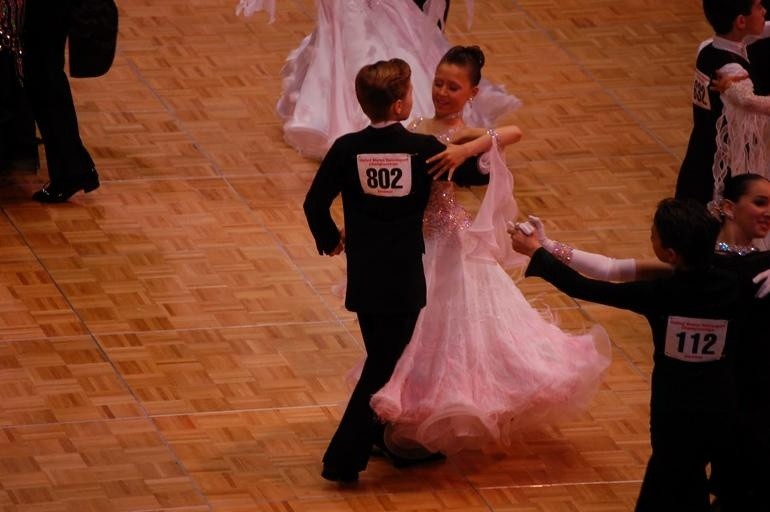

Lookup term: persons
[303,57,491,483]
[233,0,523,164]
[0,0,101,203]
[337,46,613,466]
[675,0,770,211]
[714,241,760,256]
[709,70,770,255]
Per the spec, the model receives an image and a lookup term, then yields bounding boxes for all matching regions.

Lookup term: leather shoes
[33,168,98,203]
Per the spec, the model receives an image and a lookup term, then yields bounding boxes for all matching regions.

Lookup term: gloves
[752,269,770,298]
[507,215,636,282]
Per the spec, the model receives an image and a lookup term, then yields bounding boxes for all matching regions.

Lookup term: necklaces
[435,111,464,119]
